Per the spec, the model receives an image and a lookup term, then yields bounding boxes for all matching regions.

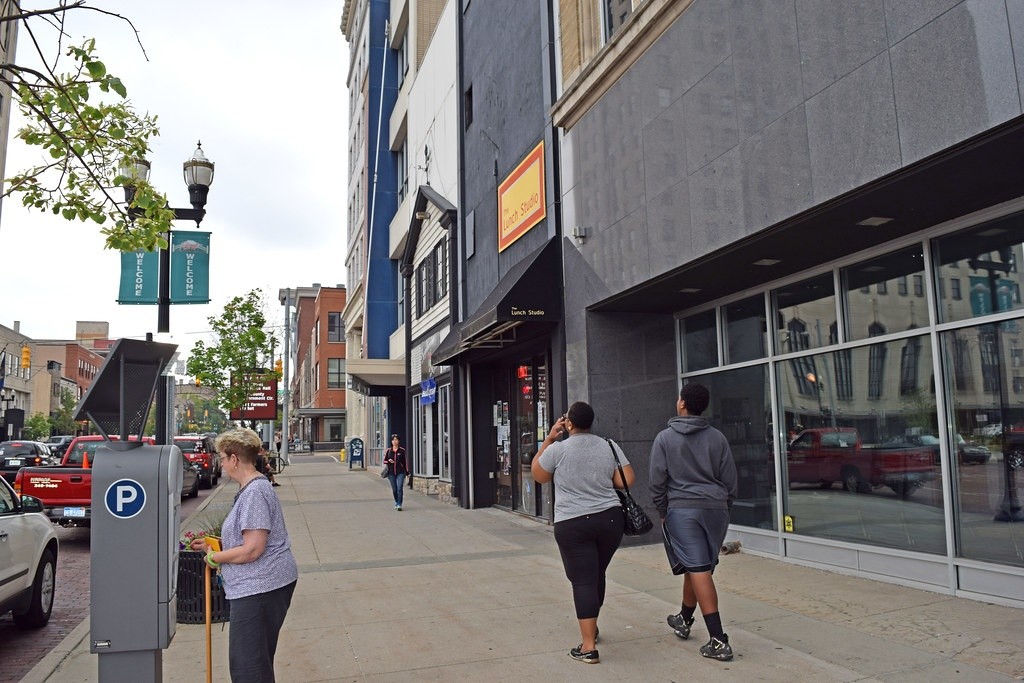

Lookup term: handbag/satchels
[381,465,389,478]
[603,437,654,536]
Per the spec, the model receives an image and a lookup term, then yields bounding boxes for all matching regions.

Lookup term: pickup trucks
[785,426,937,498]
[13,433,155,529]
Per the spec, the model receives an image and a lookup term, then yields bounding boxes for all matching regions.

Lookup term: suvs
[173,435,219,489]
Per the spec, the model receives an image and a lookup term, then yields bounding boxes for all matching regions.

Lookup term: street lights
[118,137,215,445]
[806,371,832,427]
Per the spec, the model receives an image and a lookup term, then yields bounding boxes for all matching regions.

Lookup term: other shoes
[397,505,402,511]
[272,482,280,487]
[395,506,398,510]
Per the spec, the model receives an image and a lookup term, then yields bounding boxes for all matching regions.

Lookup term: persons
[956,427,967,481]
[530,402,636,665]
[189,428,298,682]
[647,383,740,660]
[310,439,314,454]
[383,434,412,511]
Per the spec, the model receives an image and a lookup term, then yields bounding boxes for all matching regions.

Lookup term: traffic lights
[195,374,200,388]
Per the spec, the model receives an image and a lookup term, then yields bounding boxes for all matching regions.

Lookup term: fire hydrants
[339,449,346,462]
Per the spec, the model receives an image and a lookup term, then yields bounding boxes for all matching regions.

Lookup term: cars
[974,420,1024,472]
[42,435,77,459]
[0,475,59,630]
[881,429,993,466]
[181,454,200,498]
[180,432,222,477]
[0,440,59,486]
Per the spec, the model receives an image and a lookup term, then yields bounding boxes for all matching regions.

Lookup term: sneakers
[666,611,695,639]
[569,643,600,664]
[699,632,733,661]
[593,626,599,643]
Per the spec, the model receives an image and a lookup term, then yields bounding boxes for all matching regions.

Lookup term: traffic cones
[82,451,90,468]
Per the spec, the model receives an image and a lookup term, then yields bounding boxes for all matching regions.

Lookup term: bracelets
[207,551,220,566]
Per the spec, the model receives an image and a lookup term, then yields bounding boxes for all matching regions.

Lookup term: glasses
[219,454,239,463]
[391,434,398,438]
[562,413,573,424]
